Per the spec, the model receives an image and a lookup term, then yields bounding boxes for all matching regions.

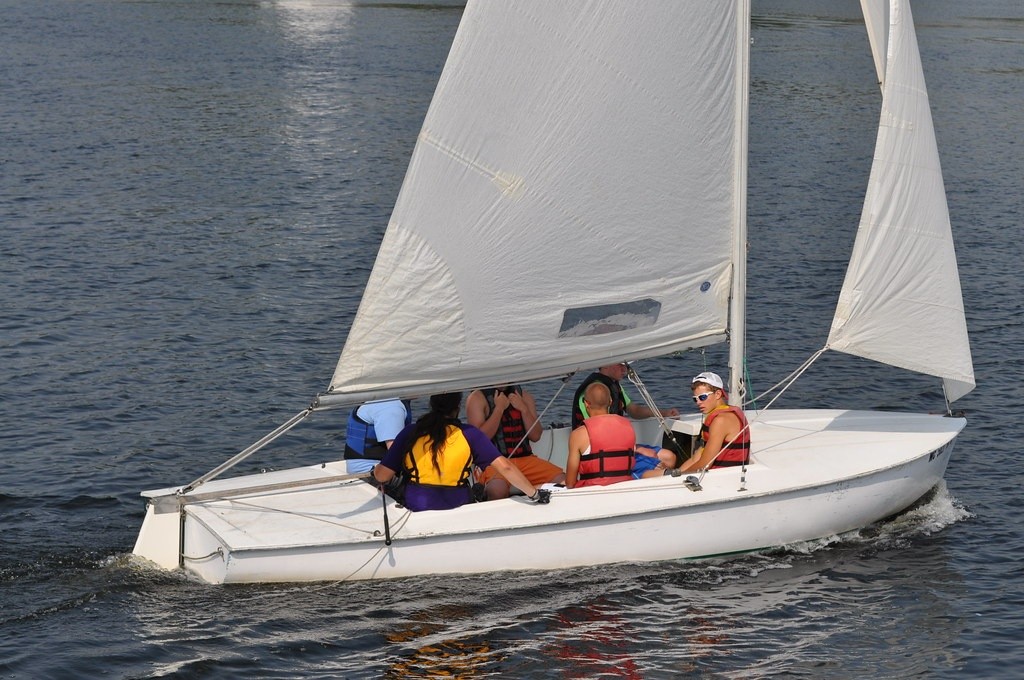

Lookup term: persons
[343,396,418,463]
[465,381,568,501]
[565,383,636,490]
[571,362,682,481]
[368,391,551,513]
[639,372,750,486]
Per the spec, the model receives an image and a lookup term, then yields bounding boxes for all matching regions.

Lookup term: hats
[692,372,730,400]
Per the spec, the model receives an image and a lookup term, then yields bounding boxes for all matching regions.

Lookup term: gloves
[531,489,551,503]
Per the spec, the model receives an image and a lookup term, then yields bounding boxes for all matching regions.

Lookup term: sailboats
[122,0,976,590]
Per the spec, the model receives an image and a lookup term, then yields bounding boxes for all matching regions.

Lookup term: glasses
[692,392,713,402]
